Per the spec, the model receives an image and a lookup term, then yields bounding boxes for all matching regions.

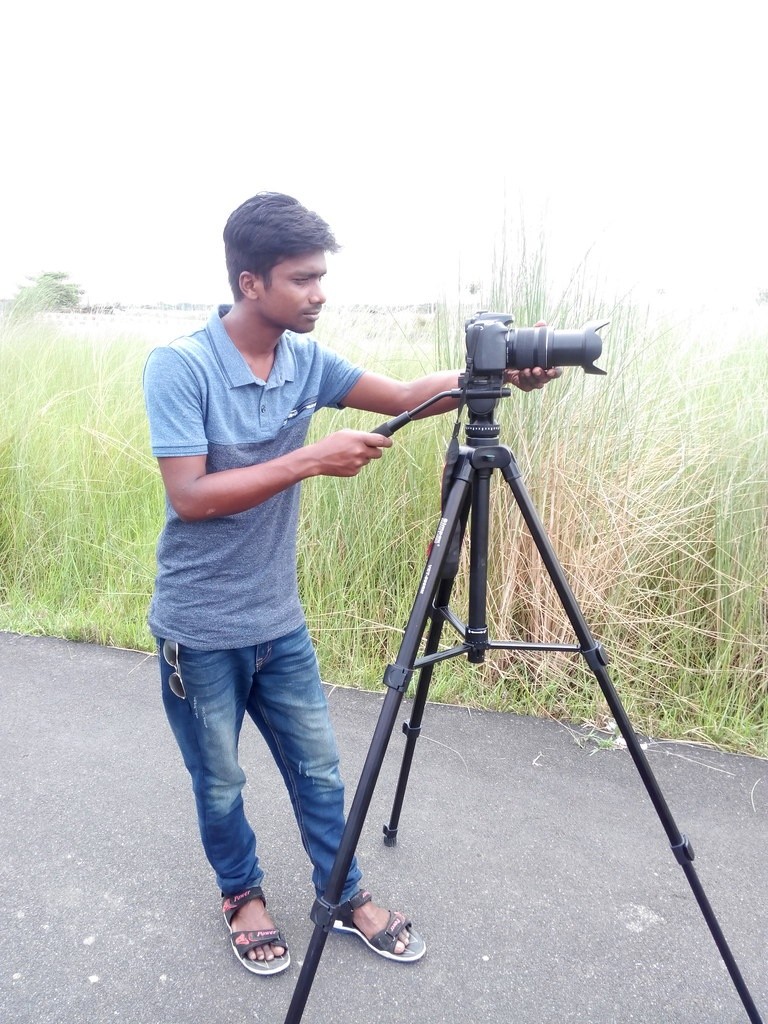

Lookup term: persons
[142,192,562,975]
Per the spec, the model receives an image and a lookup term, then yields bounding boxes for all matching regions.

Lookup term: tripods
[282,376,764,1024]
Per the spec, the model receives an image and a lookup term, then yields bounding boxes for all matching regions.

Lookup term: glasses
[163,639,186,699]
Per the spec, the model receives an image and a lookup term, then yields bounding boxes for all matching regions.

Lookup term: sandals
[331,888,426,962]
[220,886,291,976]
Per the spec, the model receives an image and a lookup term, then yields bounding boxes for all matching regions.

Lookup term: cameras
[464,311,612,378]
